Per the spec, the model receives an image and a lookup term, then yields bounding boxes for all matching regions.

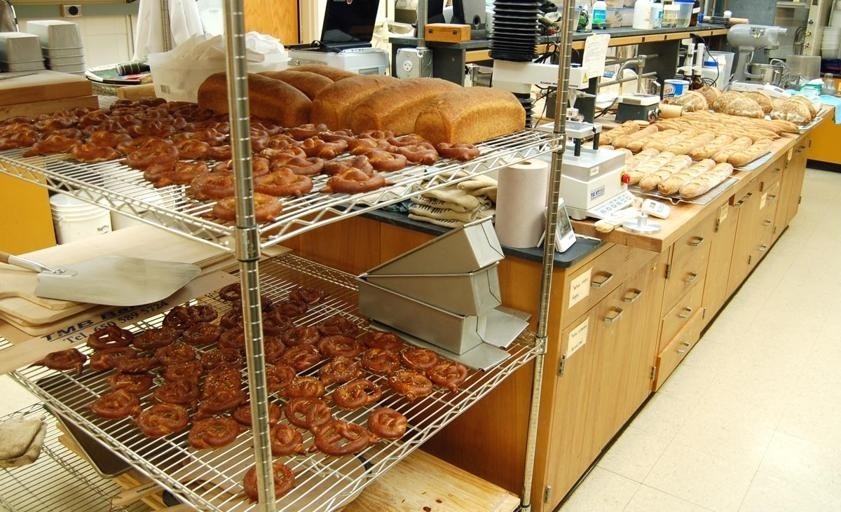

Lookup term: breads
[348,77,464,137]
[288,64,359,82]
[257,70,335,101]
[414,87,526,146]
[594,82,818,201]
[197,72,311,129]
[310,74,401,132]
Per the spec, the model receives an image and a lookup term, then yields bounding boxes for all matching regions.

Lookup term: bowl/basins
[822,26,841,60]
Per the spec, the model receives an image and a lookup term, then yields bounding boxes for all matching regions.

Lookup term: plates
[86,68,150,84]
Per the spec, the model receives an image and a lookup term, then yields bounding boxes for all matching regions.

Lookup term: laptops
[284,0,380,51]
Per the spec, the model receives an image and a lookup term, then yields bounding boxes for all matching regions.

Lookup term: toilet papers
[494,157,551,249]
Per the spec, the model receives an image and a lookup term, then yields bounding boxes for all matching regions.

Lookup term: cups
[639,75,662,95]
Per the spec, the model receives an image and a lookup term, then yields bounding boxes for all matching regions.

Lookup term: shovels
[0,250,205,308]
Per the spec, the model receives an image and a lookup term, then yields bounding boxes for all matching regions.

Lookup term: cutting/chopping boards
[2,224,239,337]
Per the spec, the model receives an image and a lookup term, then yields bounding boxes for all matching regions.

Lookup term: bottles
[724,9,733,19]
[662,1,675,28]
[578,9,589,30]
[592,0,608,29]
[702,61,721,89]
[690,0,701,26]
[823,73,837,106]
[684,66,704,88]
[653,0,663,29]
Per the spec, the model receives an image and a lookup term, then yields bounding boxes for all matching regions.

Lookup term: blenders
[725,22,796,100]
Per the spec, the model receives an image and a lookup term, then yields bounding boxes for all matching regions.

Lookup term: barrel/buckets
[50,189,113,245]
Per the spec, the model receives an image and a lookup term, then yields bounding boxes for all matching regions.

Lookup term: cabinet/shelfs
[275,106,832,512]
[0,0,574,512]
